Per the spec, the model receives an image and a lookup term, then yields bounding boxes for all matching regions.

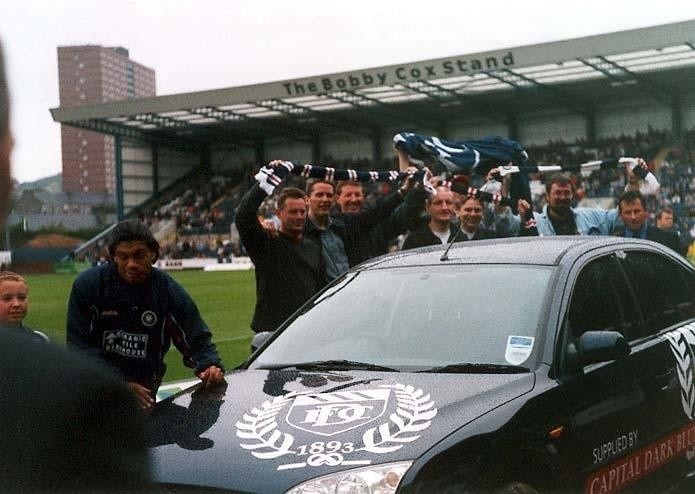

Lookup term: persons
[262,367,357,399]
[81,238,111,269]
[236,158,328,359]
[257,191,281,230]
[336,124,695,270]
[0,35,151,492]
[259,173,418,300]
[151,164,249,264]
[63,219,225,409]
[148,379,229,450]
[0,269,51,341]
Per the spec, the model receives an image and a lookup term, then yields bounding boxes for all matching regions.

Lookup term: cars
[99,234,693,494]
